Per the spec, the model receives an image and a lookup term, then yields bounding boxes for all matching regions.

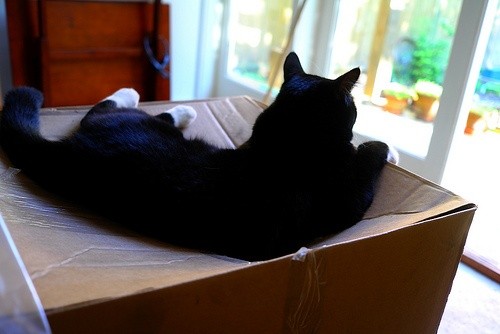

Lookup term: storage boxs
[1,93,479,334]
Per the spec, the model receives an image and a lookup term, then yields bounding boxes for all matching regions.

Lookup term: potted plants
[414,78,443,123]
[380,81,414,114]
[464,110,483,135]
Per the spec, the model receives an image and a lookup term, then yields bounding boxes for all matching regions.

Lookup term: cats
[0,51,399,262]
